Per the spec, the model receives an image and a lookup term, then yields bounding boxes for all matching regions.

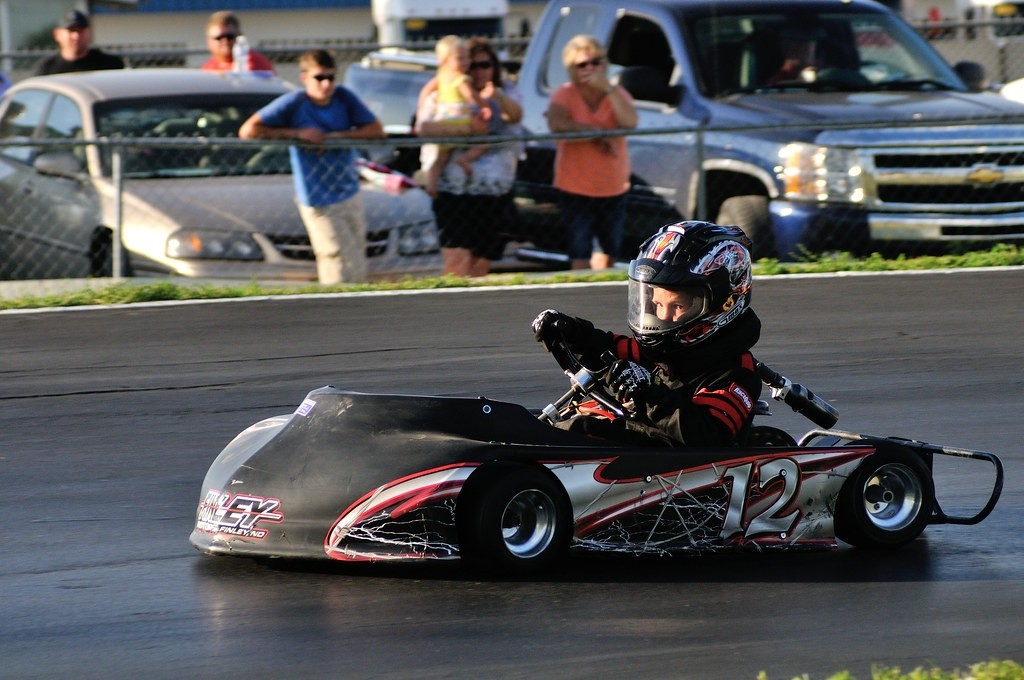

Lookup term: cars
[336,47,525,169]
[0,66,446,286]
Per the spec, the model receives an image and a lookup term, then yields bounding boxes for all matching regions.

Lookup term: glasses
[208,32,237,42]
[571,54,603,70]
[300,73,335,84]
[468,59,494,70]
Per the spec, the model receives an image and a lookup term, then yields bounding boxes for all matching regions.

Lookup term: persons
[415,32,525,276]
[27,9,124,77]
[239,49,386,286]
[532,221,762,451]
[200,10,272,71]
[548,35,635,271]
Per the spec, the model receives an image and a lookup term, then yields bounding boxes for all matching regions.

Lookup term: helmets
[627,221,751,355]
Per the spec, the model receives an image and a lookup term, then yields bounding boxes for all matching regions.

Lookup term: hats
[56,11,89,33]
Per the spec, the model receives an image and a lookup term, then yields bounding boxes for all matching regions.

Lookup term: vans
[367,2,514,80]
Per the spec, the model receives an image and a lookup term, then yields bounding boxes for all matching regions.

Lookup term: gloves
[607,361,658,413]
[533,309,593,354]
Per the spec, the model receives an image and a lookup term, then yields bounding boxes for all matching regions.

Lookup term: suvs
[513,1,1024,254]
